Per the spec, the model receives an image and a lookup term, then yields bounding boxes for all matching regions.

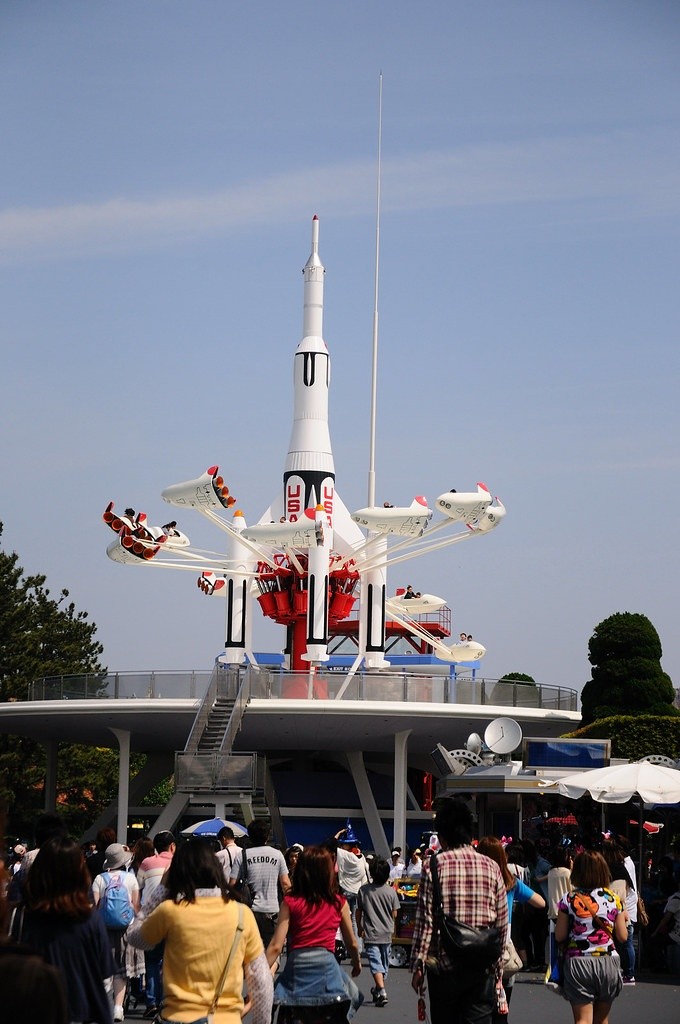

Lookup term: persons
[124,508,136,525]
[404,585,421,599]
[383,502,394,508]
[0,800,680,1024]
[160,521,178,537]
[280,517,289,523]
[450,489,457,493]
[456,633,472,646]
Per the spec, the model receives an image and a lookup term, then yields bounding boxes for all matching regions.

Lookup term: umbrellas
[538,763,680,970]
[179,817,250,838]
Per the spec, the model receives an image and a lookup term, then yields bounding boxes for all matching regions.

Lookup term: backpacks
[99,871,134,929]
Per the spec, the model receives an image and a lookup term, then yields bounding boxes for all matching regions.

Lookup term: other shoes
[113,1006,124,1021]
[374,995,388,1007]
[371,987,379,1002]
[143,1004,158,1018]
[622,978,635,986]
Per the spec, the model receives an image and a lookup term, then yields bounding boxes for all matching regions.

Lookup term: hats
[102,844,133,870]
[366,854,373,861]
[338,817,357,842]
[391,851,400,856]
[14,845,27,855]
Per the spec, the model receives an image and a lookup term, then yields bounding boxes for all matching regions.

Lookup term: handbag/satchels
[432,914,503,968]
[494,933,524,973]
[636,895,649,926]
[228,846,253,909]
[493,967,509,1016]
